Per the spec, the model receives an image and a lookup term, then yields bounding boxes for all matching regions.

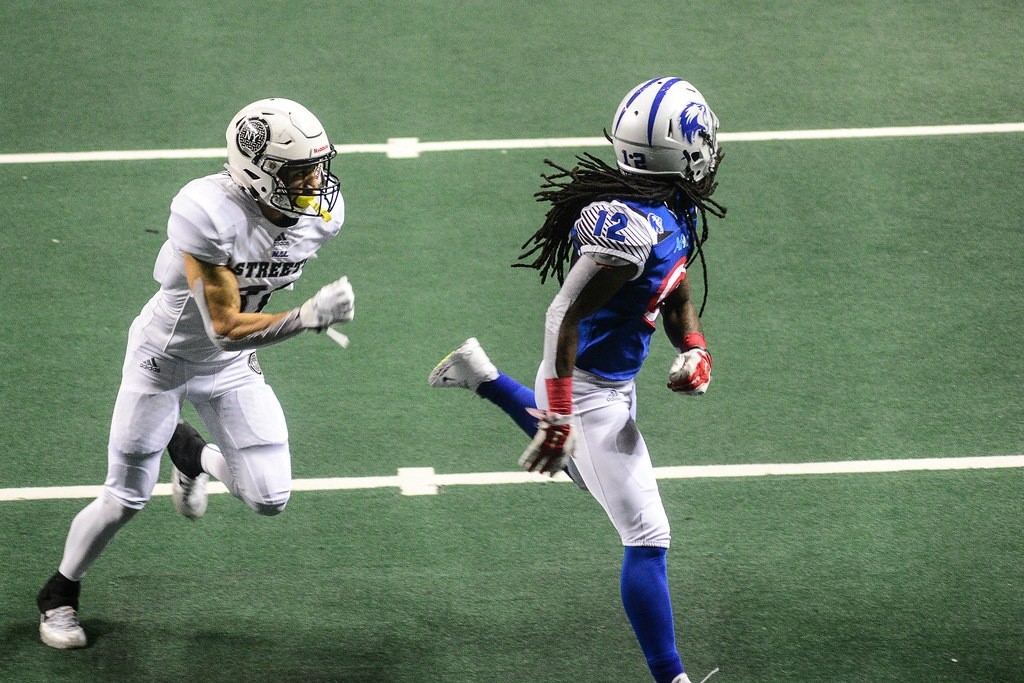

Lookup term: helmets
[226,97,339,221]
[612,75,720,185]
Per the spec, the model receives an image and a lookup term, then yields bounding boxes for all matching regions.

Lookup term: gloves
[298,274,355,334]
[520,404,575,477]
[667,347,713,396]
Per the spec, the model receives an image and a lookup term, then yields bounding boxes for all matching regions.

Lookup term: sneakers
[429,338,502,399]
[37,569,87,650]
[168,418,210,518]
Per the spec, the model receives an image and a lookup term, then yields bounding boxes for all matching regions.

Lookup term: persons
[429,77,726,683]
[37,97,354,649]
